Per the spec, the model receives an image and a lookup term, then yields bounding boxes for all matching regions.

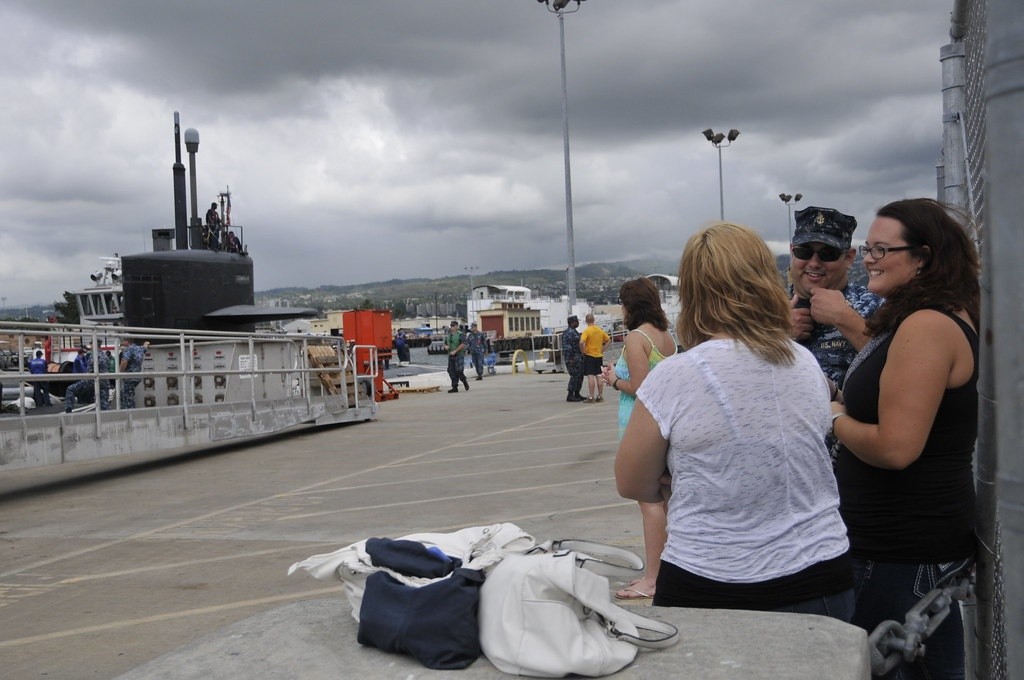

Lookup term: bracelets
[832,387,838,401]
[612,378,622,391]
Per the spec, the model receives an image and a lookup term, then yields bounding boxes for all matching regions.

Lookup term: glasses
[859,244,916,260]
[793,243,846,262]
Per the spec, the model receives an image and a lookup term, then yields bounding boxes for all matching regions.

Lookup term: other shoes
[595,396,603,402]
[584,396,595,402]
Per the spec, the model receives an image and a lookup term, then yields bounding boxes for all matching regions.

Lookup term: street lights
[702,127,741,220]
[537,0,588,309]
[779,193,804,265]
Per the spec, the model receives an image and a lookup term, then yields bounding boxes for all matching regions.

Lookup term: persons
[62,333,150,413]
[579,314,612,403]
[562,315,588,402]
[777,199,988,680]
[29,350,54,407]
[442,321,470,393]
[600,277,679,600]
[466,322,488,380]
[395,328,408,361]
[614,223,856,623]
[205,202,241,253]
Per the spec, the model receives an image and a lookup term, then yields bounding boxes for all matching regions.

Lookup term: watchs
[830,412,845,435]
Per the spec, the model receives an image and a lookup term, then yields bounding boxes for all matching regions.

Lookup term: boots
[463,380,469,390]
[575,390,587,400]
[566,389,581,402]
[476,373,482,380]
[448,385,458,393]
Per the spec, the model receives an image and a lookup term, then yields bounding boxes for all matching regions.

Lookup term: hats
[450,321,458,325]
[90,338,102,346]
[470,323,477,327]
[792,207,858,252]
[567,316,582,322]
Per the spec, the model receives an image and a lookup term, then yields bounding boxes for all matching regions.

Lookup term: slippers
[615,586,655,599]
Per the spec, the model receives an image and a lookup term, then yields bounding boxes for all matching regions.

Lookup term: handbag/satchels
[287,521,680,680]
